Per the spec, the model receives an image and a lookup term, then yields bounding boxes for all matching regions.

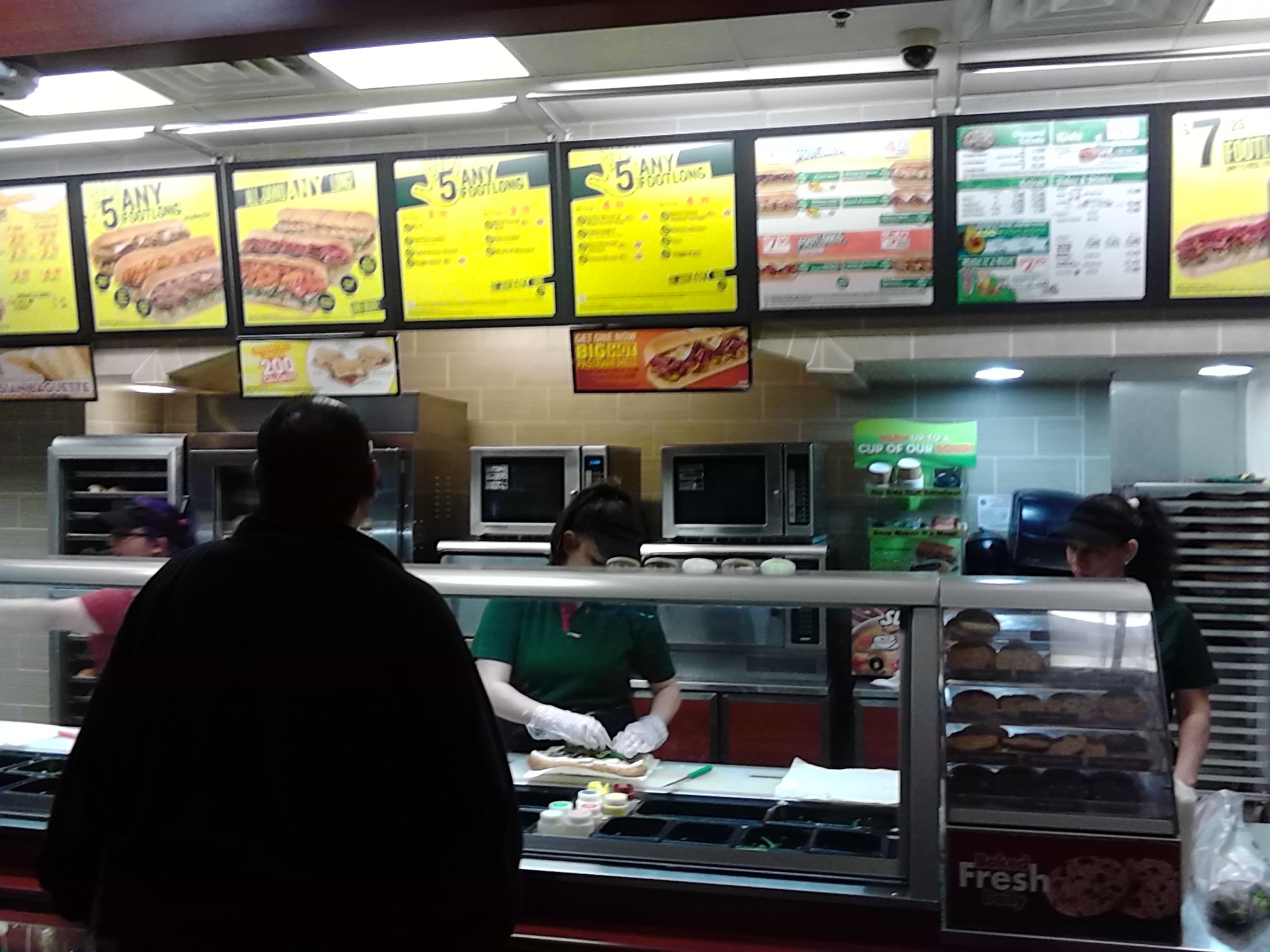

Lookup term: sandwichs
[754,165,801,283]
[90,206,377,325]
[642,329,751,388]
[1174,211,1269,280]
[310,343,392,386]
[887,162,932,278]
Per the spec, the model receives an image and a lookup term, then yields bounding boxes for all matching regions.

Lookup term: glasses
[109,528,148,538]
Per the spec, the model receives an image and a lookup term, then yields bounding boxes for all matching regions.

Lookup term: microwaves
[465,445,641,542]
[657,436,821,544]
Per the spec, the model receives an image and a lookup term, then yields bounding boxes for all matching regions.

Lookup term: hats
[1053,493,1144,548]
[94,498,181,534]
[574,508,649,560]
[250,393,374,524]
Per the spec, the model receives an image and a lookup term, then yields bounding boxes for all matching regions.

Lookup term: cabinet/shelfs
[850,692,903,773]
[630,680,828,770]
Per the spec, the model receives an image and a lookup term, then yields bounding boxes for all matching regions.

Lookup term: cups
[604,555,797,576]
[865,455,926,495]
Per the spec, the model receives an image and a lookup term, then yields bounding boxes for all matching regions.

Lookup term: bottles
[537,786,628,836]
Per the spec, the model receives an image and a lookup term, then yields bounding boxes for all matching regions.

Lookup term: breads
[944,608,1153,806]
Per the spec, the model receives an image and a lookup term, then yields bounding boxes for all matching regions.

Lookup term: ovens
[186,391,468,575]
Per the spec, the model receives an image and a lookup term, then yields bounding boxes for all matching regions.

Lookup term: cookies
[1045,854,1179,922]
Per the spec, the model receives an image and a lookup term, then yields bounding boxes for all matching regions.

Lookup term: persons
[466,482,685,763]
[38,390,525,952]
[0,497,200,681]
[1063,493,1219,794]
